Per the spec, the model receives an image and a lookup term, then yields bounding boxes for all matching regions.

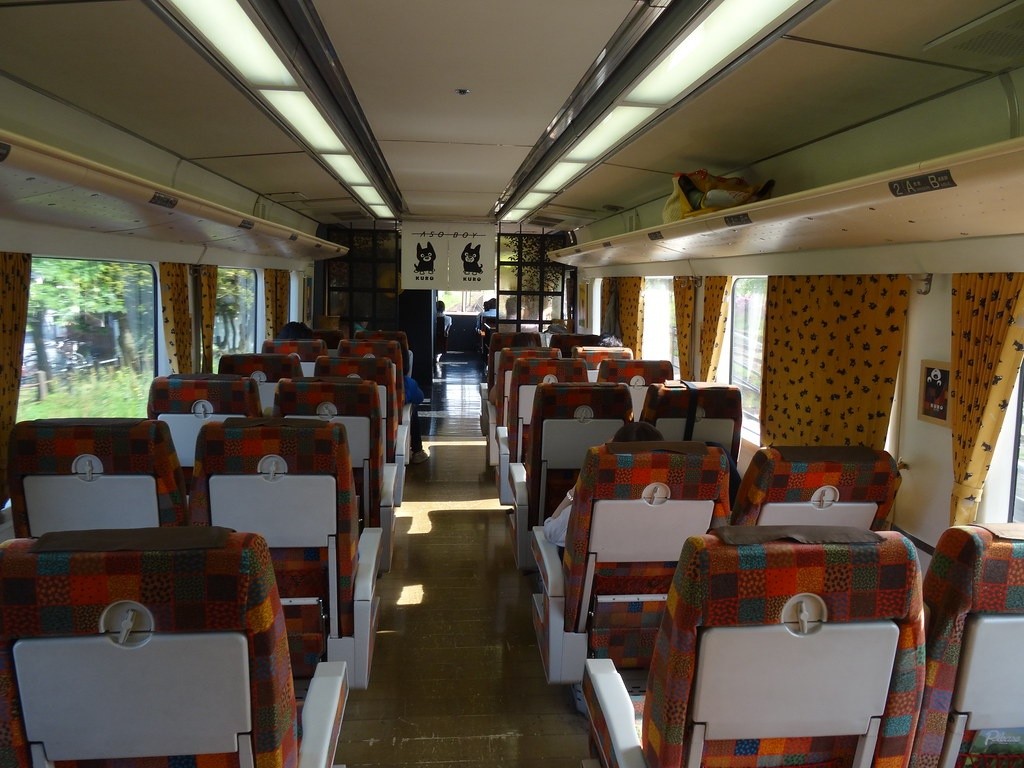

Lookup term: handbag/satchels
[662,168,776,224]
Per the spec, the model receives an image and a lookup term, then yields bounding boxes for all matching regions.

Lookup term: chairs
[475,315,1024,768]
[0,316,449,768]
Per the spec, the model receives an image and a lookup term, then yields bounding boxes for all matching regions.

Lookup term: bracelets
[566,490,574,505]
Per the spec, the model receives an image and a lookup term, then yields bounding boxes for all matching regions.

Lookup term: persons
[436,300,452,332]
[598,333,623,347]
[476,298,567,334]
[543,422,664,567]
[279,322,316,339]
[400,343,430,463]
[488,333,542,405]
[354,313,368,334]
